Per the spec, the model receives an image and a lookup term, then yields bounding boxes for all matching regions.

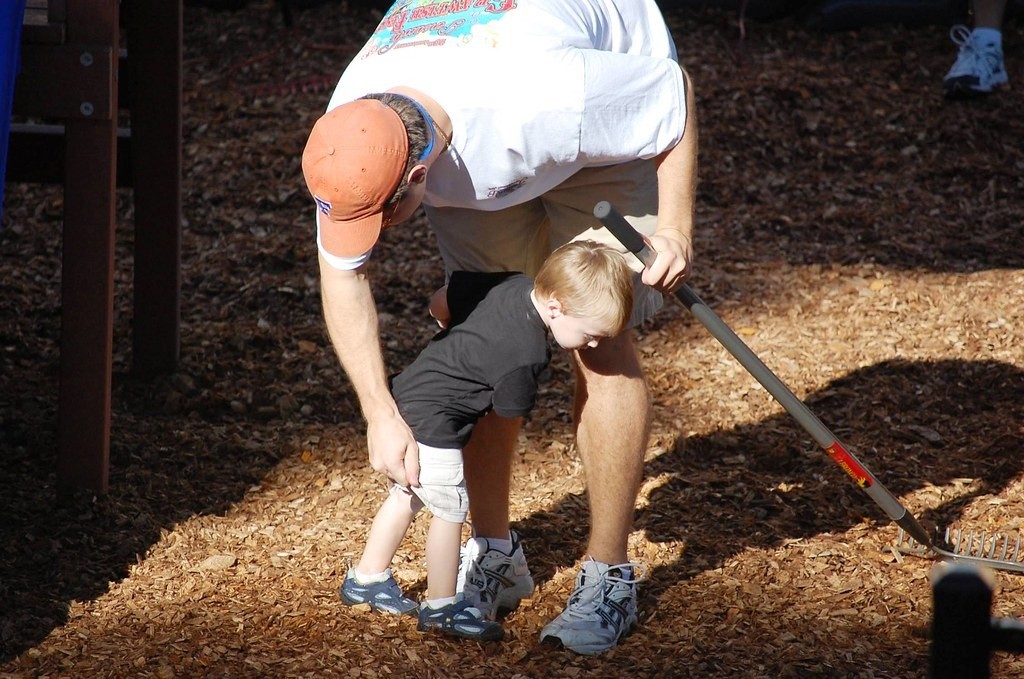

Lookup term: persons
[942,0,1009,95]
[339,240,635,642]
[301,0,699,657]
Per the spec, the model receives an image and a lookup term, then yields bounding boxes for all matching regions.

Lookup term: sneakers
[943,25,1009,91]
[418,593,505,641]
[340,569,419,615]
[456,531,535,617]
[539,555,645,654]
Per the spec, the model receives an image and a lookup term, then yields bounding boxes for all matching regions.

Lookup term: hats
[300,101,407,258]
